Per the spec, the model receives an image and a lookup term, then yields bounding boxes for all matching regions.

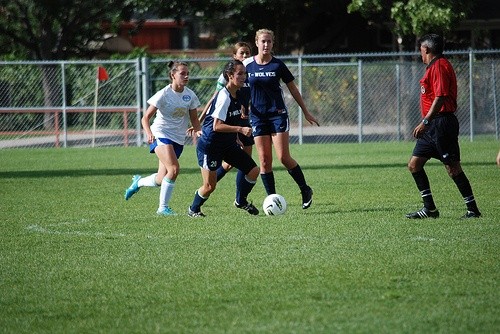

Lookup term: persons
[188,60,261,217]
[185,42,255,182]
[125,61,201,216]
[242,29,320,210]
[405,34,481,219]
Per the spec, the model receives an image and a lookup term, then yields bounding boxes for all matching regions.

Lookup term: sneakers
[461,212,481,219]
[188,205,205,219]
[234,199,259,215]
[125,175,141,201]
[406,206,439,218]
[301,186,313,209]
[157,207,176,216]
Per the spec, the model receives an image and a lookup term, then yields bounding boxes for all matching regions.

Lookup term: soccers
[264,194,286,215]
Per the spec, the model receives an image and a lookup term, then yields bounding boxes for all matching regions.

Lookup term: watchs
[423,118,428,125]
